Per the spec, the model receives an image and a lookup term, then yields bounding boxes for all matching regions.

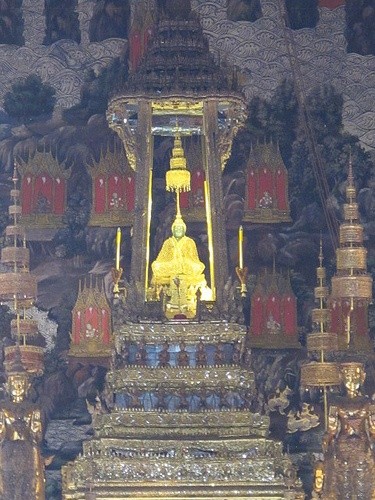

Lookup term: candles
[116,227,121,272]
[238,224,245,270]
